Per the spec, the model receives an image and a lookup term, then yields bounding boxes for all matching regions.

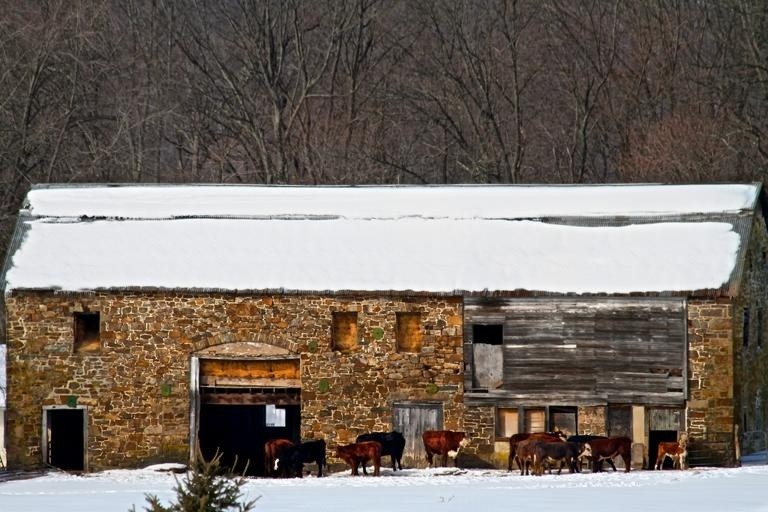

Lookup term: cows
[507,429,633,476]
[355,430,406,475]
[422,429,472,468]
[272,438,327,477]
[331,440,382,477]
[653,432,690,470]
[263,438,296,477]
